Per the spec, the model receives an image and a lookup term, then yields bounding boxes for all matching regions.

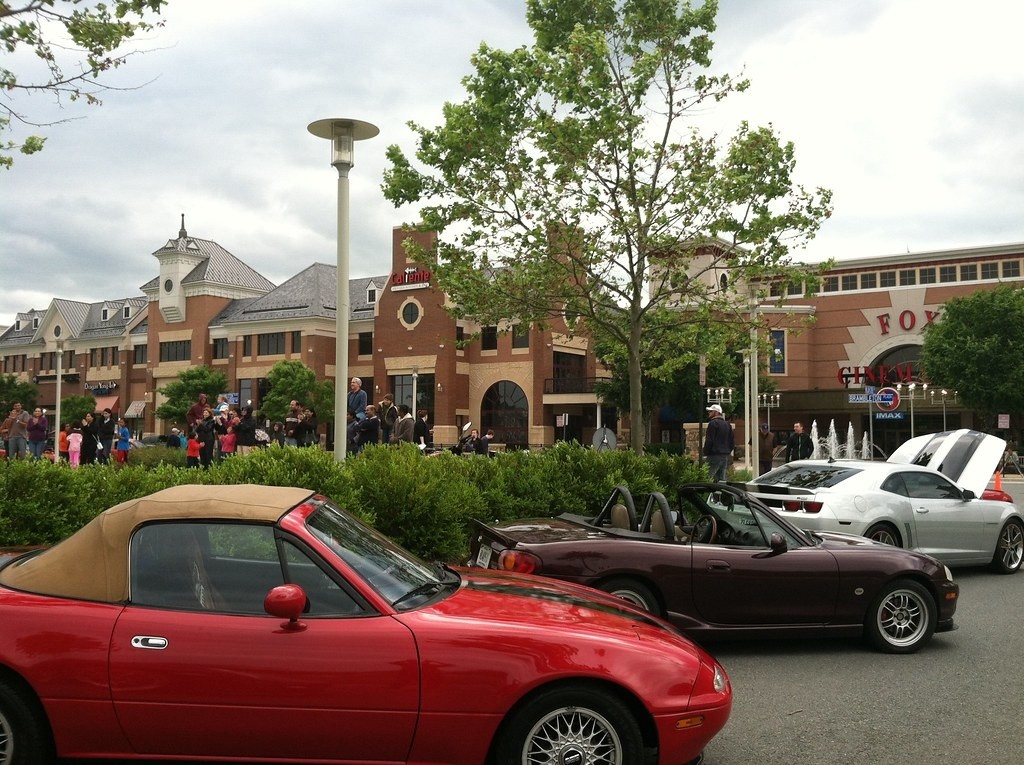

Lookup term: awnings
[93,396,120,414]
[124,401,146,418]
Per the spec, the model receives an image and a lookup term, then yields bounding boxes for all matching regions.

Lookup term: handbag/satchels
[97,442,104,450]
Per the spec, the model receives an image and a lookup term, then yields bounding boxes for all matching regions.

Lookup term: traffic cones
[994,471,1002,491]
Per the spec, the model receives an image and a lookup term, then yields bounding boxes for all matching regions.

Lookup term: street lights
[307,118,380,463]
[48,338,71,464]
[737,349,752,470]
[739,283,773,477]
[407,365,420,419]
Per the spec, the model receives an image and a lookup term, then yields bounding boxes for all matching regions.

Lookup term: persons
[166,394,320,470]
[748,424,777,476]
[0,400,49,466]
[703,404,734,481]
[58,408,130,469]
[347,377,435,455]
[785,420,815,464]
[461,429,496,455]
[1003,448,1020,473]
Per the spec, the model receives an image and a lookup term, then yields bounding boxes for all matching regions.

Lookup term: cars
[977,489,1014,504]
[139,434,169,448]
[110,439,145,455]
[702,429,1024,576]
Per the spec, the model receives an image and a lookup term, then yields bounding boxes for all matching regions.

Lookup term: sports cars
[1,483,733,765]
[462,480,961,654]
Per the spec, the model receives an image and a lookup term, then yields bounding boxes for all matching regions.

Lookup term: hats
[219,405,229,412]
[706,404,722,414]
[383,394,394,401]
[171,428,180,433]
[761,423,768,432]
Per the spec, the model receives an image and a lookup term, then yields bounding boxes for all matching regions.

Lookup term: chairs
[610,504,633,531]
[651,509,697,542]
